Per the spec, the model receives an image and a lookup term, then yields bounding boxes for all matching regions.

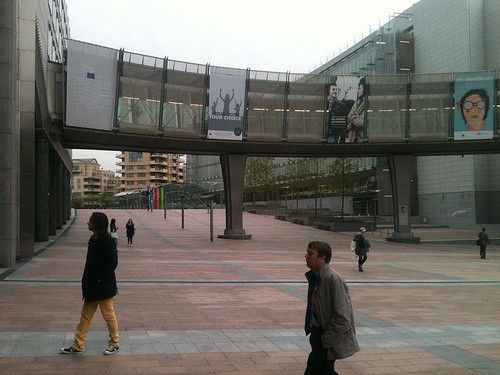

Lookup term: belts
[310,326,323,330]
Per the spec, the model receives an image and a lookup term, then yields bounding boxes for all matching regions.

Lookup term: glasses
[463,100,485,110]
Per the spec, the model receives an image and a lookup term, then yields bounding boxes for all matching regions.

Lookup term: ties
[305,276,317,336]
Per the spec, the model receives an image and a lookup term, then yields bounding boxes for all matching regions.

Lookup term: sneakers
[104,347,119,354]
[60,346,82,354]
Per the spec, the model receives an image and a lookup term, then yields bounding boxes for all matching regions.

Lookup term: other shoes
[359,265,364,272]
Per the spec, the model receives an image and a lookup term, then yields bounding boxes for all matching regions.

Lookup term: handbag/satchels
[111,229,118,239]
[351,241,356,252]
[477,240,481,246]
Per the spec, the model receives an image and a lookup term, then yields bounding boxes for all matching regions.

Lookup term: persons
[347,80,365,142]
[478,228,489,259]
[110,219,117,245]
[126,219,135,246]
[60,213,120,354]
[304,240,360,375]
[327,84,346,143]
[353,227,368,272]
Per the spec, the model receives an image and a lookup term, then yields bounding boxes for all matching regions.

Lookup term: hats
[360,227,366,233]
